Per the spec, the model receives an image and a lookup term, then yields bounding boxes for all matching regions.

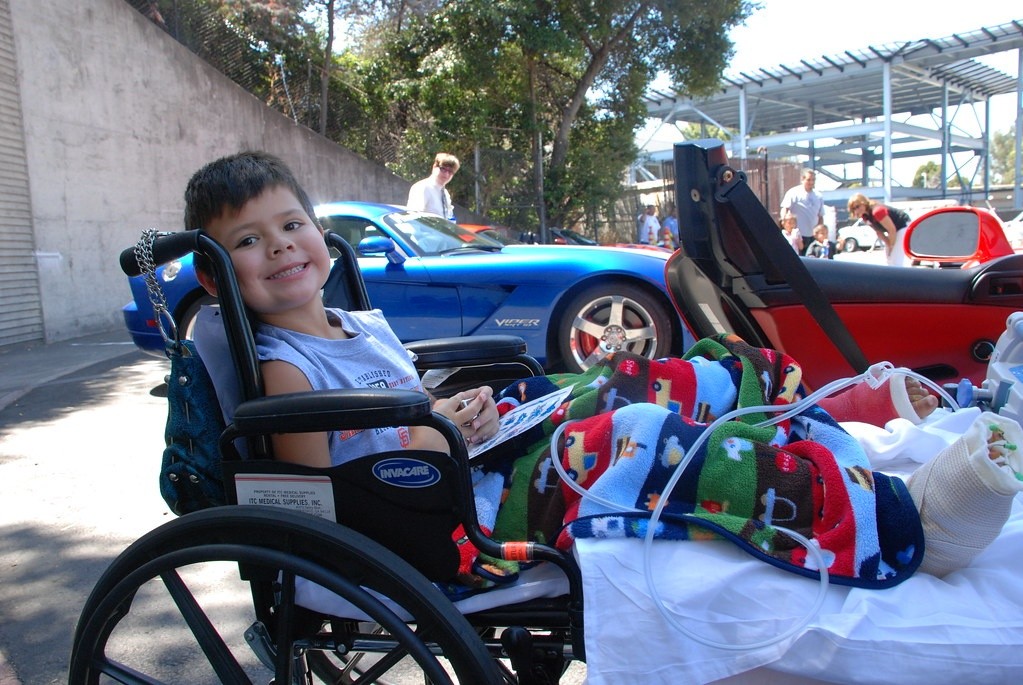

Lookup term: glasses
[435,166,455,176]
[850,204,863,211]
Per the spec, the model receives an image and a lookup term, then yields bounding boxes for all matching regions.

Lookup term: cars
[662,128,1023,412]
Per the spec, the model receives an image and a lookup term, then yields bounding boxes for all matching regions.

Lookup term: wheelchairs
[60,217,654,684]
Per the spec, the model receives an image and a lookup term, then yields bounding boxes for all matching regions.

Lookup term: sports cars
[121,200,712,383]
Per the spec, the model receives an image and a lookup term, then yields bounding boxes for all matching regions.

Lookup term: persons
[635,203,663,246]
[406,152,460,223]
[804,225,844,258]
[663,205,680,249]
[182,146,1023,580]
[778,167,825,256]
[779,213,803,254]
[845,194,912,269]
